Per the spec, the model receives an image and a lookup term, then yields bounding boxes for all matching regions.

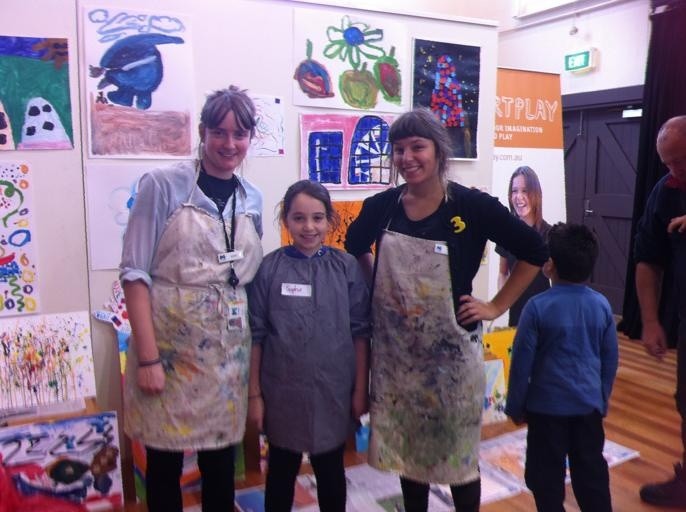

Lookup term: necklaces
[201,171,241,289]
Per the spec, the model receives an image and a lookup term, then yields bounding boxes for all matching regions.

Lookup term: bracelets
[248,393,263,399]
[138,358,162,367]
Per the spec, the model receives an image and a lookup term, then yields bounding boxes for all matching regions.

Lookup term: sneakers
[638,461,686,509]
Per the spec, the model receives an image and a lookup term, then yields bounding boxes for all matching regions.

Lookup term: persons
[243,179,374,512]
[500,219,622,511]
[115,87,266,512]
[635,114,686,509]
[491,165,556,328]
[668,215,686,235]
[343,106,551,511]
